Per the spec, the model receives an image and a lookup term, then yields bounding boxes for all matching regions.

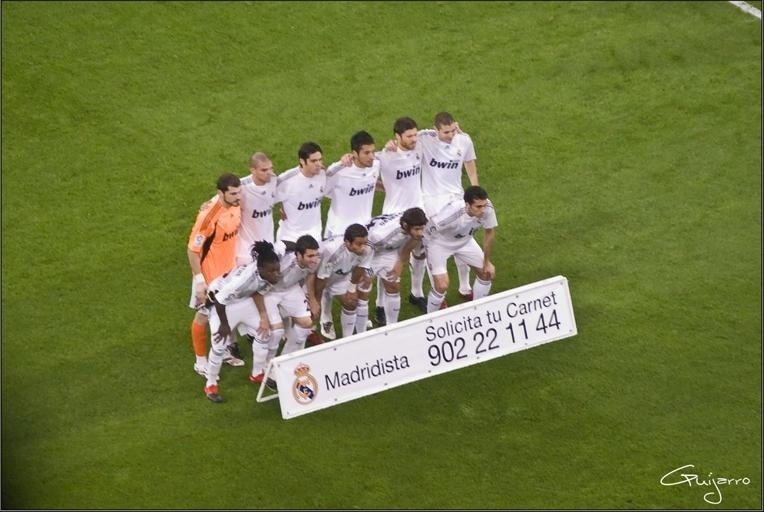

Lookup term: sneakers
[374,299,386,324]
[193,363,220,381]
[308,330,325,345]
[223,334,255,367]
[439,299,448,310]
[249,372,277,392]
[203,384,222,403]
[409,291,428,312]
[319,316,336,340]
[367,319,373,328]
[457,287,473,300]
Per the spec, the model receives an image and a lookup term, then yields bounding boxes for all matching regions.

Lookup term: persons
[279,128,386,341]
[354,206,429,335]
[275,140,327,347]
[339,118,468,326]
[423,186,501,315]
[186,171,245,383]
[250,234,322,393]
[202,239,281,404]
[384,112,480,313]
[315,222,374,340]
[238,150,278,267]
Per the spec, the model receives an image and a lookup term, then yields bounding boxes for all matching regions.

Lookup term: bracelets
[194,273,205,284]
